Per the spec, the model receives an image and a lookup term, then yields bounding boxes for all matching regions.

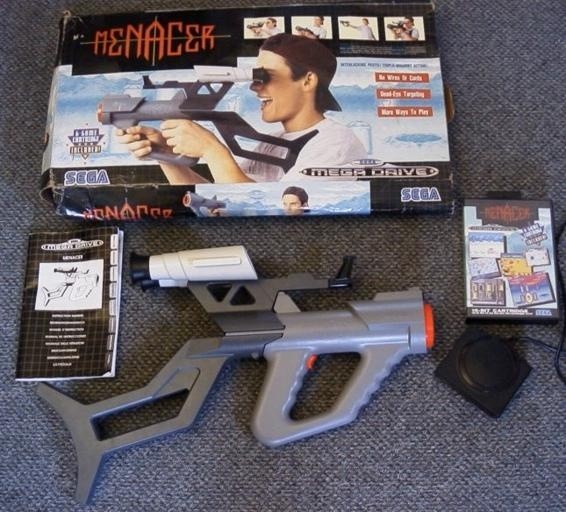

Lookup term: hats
[319,16,324,24]
[283,187,310,213]
[268,17,276,27]
[263,33,342,112]
[364,19,369,24]
[405,16,414,26]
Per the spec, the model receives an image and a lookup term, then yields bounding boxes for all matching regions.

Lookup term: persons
[113,33,367,185]
[298,16,326,39]
[250,18,279,39]
[392,16,418,41]
[206,187,310,217]
[341,17,375,39]
